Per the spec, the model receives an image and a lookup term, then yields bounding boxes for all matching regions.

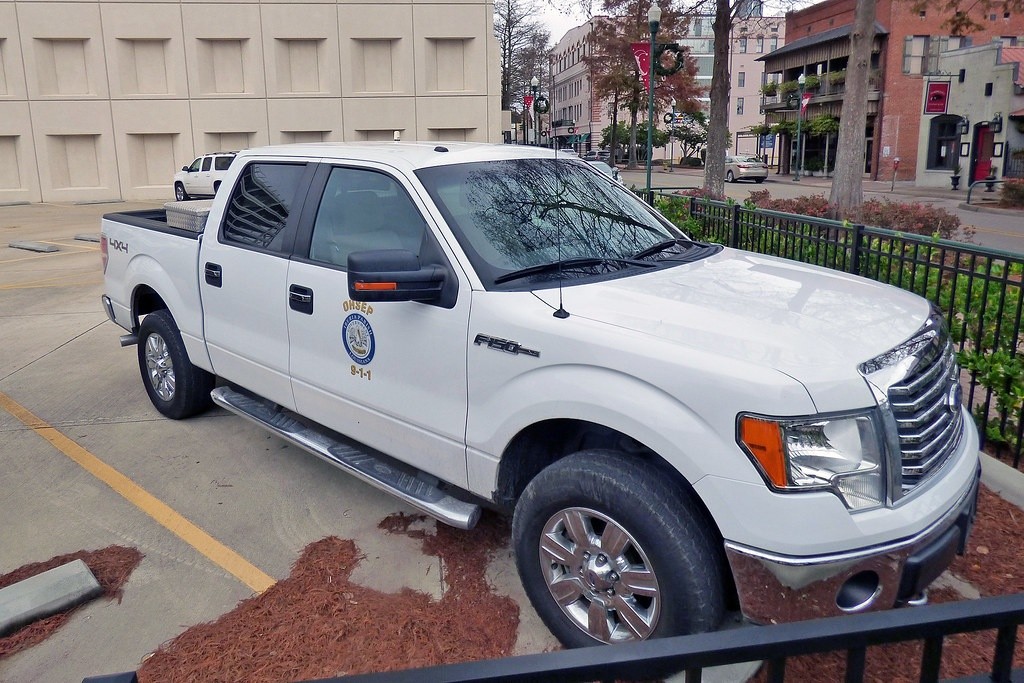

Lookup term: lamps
[989,110,1003,133]
[957,114,970,134]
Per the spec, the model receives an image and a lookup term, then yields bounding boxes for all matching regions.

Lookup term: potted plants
[779,80,800,95]
[828,71,845,85]
[804,75,820,89]
[950,162,964,191]
[760,81,778,96]
[984,166,999,192]
[744,123,770,136]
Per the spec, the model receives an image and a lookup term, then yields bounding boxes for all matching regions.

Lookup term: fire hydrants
[663,161,668,170]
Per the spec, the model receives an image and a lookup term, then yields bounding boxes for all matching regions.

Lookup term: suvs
[582,151,610,161]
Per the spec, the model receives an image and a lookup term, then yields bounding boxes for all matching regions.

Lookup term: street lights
[531,76,539,145]
[795,74,806,181]
[647,2,662,206]
[670,99,677,170]
[573,119,575,150]
[610,113,613,148]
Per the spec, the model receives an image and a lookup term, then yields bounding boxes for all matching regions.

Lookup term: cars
[587,161,623,185]
[723,156,769,183]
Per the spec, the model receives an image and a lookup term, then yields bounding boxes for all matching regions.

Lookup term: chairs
[456,171,512,262]
[330,191,403,265]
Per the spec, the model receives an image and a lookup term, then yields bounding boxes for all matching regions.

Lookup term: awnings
[568,133,590,143]
[548,138,552,144]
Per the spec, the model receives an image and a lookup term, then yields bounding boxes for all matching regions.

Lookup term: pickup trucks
[174,152,238,201]
[559,149,580,158]
[101,141,982,649]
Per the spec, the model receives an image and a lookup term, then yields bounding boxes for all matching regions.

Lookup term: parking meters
[891,157,900,192]
